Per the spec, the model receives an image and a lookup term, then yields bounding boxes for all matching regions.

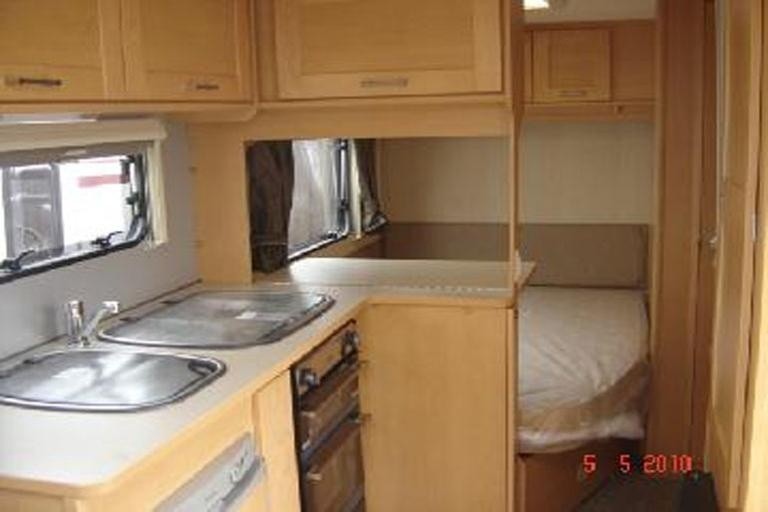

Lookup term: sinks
[1,345,227,413]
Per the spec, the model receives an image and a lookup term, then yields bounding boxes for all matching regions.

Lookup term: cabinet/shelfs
[1,1,120,120]
[270,1,504,108]
[118,0,257,119]
[532,23,656,117]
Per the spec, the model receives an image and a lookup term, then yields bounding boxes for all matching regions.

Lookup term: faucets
[62,299,121,348]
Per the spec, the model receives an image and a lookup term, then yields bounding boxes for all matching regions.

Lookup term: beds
[519,283,651,457]
